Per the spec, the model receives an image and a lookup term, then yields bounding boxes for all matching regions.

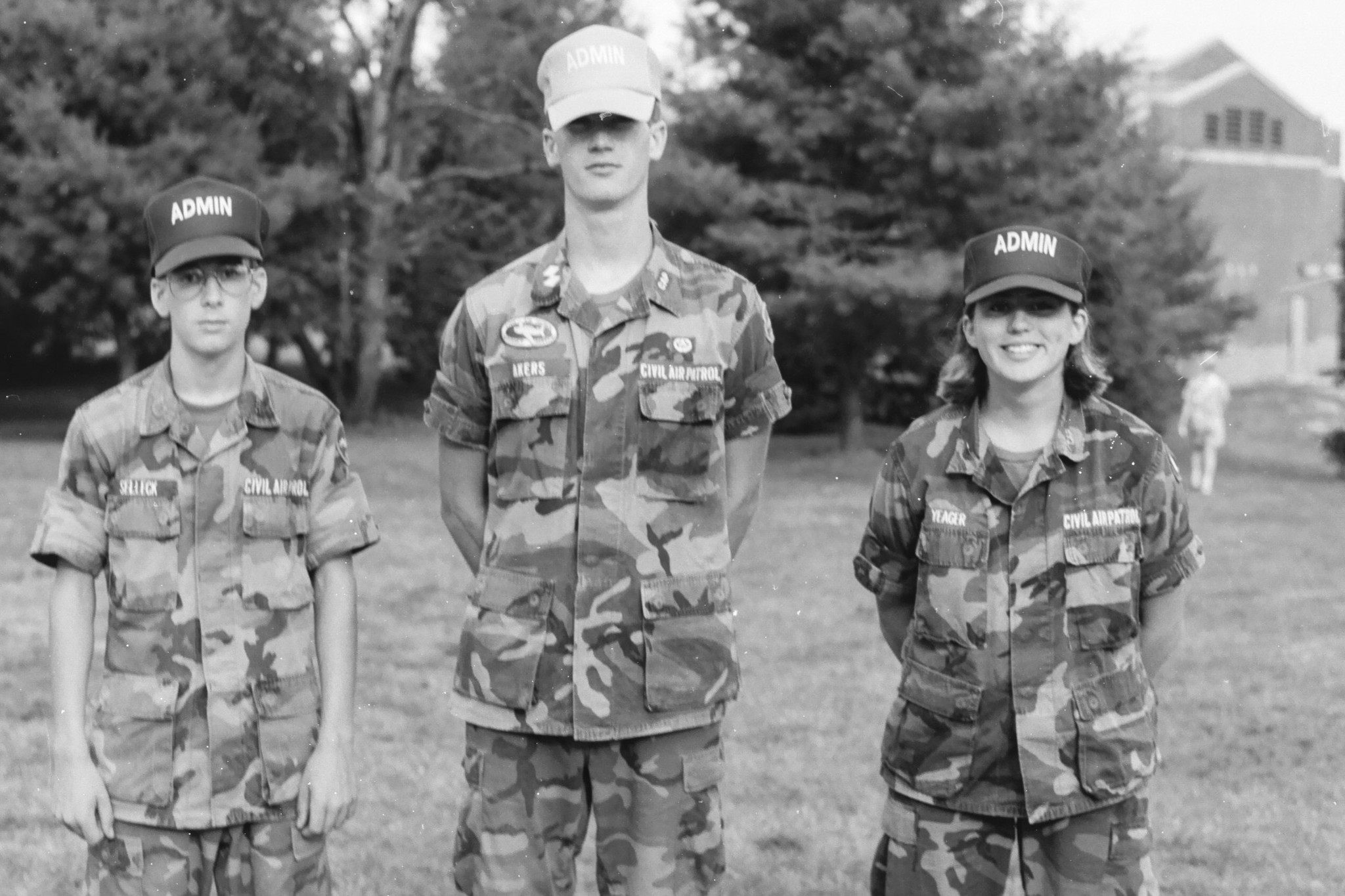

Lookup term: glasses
[158,260,254,302]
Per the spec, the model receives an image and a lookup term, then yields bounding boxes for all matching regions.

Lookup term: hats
[961,226,1088,306]
[143,174,269,281]
[536,24,660,132]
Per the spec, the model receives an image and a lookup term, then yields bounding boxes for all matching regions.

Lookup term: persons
[1179,352,1230,494]
[422,25,792,896]
[853,225,1206,896]
[32,177,381,896]
